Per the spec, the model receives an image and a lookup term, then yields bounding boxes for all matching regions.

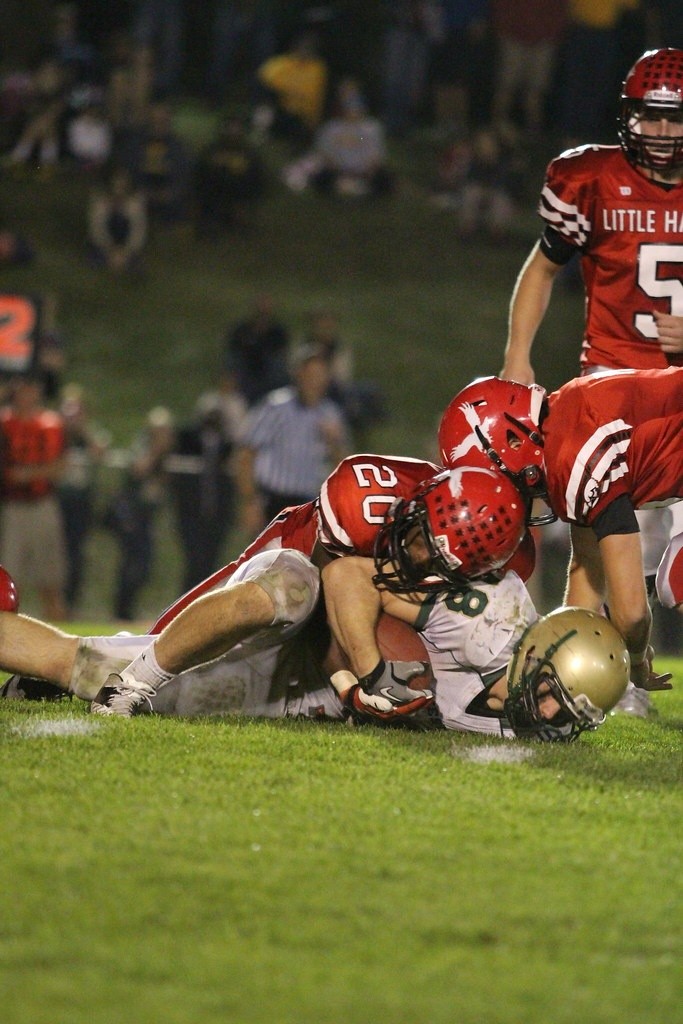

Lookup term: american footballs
[338,612,433,706]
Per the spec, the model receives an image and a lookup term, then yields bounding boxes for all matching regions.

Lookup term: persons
[0,547,630,737]
[498,49,683,713]
[148,453,524,634]
[0,0,683,622]
[435,365,683,692]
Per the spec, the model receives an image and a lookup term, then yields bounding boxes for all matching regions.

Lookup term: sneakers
[91,674,158,721]
[0,672,25,703]
[620,676,651,718]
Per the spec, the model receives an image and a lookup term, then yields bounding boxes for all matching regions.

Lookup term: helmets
[506,604,631,732]
[619,46,683,116]
[406,465,527,586]
[439,378,545,487]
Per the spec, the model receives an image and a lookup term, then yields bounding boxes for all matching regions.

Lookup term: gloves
[631,643,673,694]
[329,669,434,721]
[365,659,436,706]
